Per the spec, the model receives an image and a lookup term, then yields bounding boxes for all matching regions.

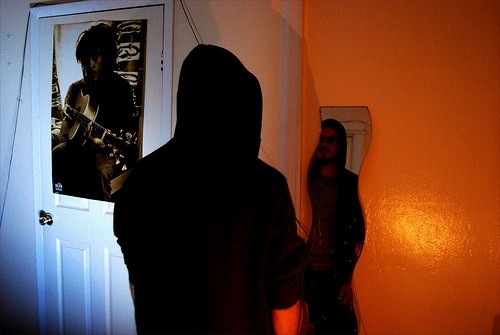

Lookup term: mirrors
[301,105,372,335]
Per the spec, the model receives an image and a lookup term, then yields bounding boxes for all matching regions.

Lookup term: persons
[301,119,366,335]
[106,43,313,335]
[47,22,142,198]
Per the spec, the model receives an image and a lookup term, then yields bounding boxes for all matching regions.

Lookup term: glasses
[319,133,335,144]
